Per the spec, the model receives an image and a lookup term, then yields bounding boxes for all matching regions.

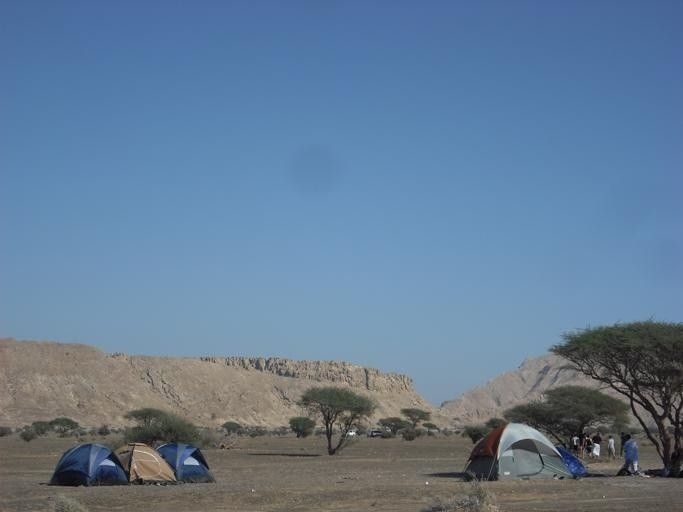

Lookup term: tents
[113,442,177,485]
[459,422,575,482]
[50,443,129,485]
[156,442,216,483]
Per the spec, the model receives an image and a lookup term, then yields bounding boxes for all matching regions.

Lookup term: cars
[373,426,390,436]
[346,429,360,437]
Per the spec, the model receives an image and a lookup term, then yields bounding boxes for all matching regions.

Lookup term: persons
[569,431,639,475]
